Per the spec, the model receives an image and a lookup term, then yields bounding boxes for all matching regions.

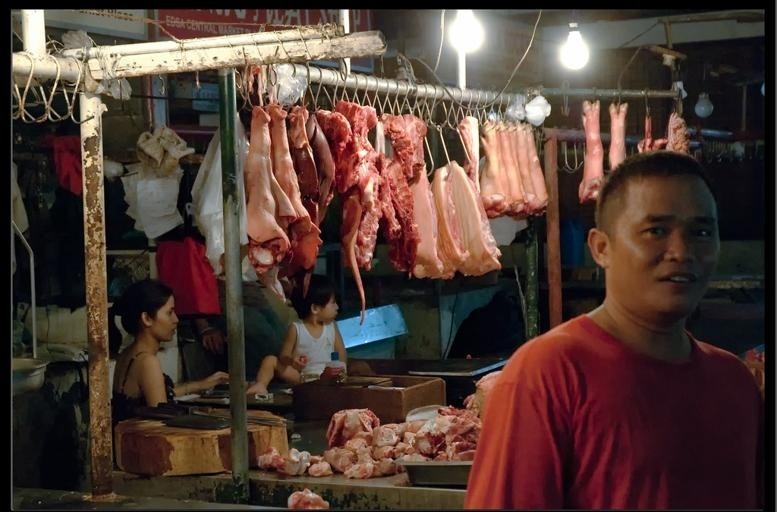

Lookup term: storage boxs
[294,376,447,423]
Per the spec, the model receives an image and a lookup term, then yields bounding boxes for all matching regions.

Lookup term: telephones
[43,344,88,362]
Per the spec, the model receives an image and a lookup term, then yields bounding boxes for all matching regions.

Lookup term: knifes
[133,409,231,430]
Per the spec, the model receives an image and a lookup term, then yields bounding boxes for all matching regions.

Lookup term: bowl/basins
[13,358,49,397]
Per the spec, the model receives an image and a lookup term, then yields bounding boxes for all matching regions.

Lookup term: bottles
[326,352,346,383]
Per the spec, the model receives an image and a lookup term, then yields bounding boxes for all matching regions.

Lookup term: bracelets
[199,326,214,336]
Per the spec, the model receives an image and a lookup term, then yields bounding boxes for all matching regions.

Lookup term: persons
[244,273,376,397]
[107,278,230,473]
[194,279,300,367]
[462,149,769,510]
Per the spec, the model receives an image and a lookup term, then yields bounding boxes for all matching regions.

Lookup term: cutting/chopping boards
[113,406,290,476]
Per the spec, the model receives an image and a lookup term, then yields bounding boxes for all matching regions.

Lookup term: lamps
[557,9,590,70]
[693,65,714,119]
[443,9,484,57]
